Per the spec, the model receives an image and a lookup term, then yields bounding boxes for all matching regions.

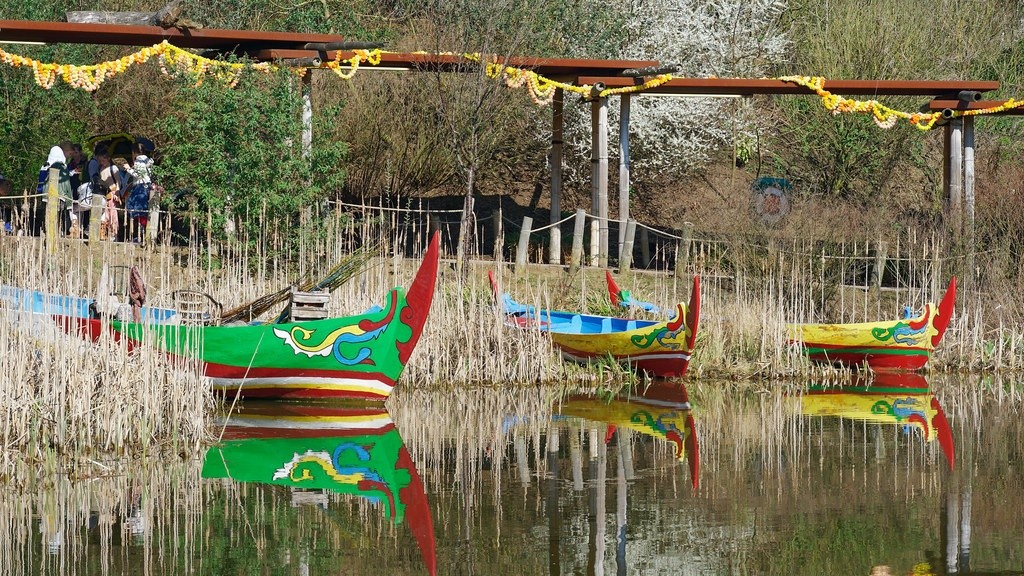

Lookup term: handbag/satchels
[77,182,107,211]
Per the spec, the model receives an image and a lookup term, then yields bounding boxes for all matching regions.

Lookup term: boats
[543,375,699,492]
[483,269,702,378]
[776,370,957,472]
[0,228,443,407]
[201,401,437,576]
[604,269,957,376]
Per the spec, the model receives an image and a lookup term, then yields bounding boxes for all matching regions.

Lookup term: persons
[37,141,164,241]
